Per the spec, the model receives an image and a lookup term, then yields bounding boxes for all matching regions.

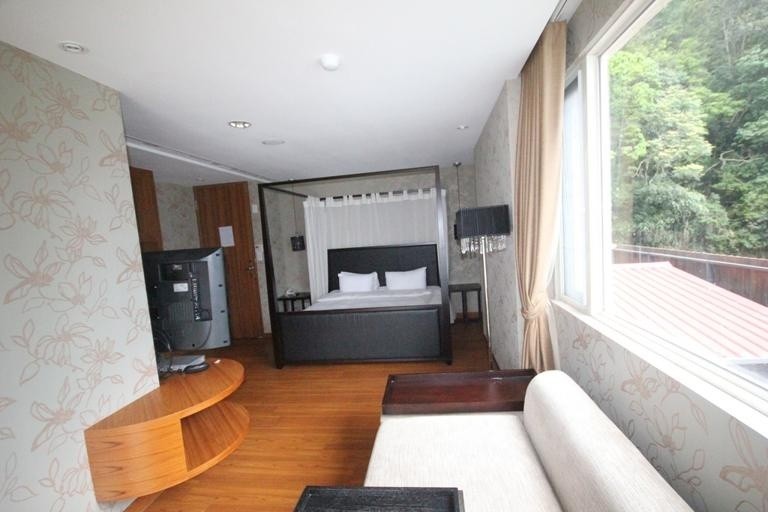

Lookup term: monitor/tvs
[140,246,231,372]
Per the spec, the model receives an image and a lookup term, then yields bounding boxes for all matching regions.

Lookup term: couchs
[364,371,696,512]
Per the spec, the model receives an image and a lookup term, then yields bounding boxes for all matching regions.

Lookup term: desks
[448,283,481,323]
[277,292,311,312]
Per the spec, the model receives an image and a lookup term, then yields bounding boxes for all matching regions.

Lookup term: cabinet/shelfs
[84,356,251,503]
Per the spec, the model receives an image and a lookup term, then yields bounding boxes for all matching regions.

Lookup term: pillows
[336,266,428,293]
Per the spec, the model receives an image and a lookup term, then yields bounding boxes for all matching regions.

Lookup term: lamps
[287,179,305,251]
[456,205,511,370]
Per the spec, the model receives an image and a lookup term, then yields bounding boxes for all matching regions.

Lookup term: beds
[275,244,444,366]
